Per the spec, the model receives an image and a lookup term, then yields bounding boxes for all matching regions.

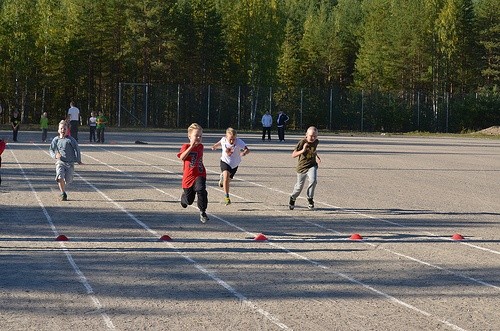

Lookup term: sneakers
[224,196,230,205]
[60,194,67,201]
[200,212,209,223]
[307,198,314,209]
[181,193,188,208]
[289,197,295,210]
[219,173,223,187]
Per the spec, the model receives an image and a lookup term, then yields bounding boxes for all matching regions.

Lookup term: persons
[176,123,210,224]
[275,109,290,144]
[89,111,97,143]
[67,101,82,144]
[211,127,249,206]
[261,110,273,142]
[40,111,49,143]
[10,111,21,143]
[65,116,71,137]
[96,110,108,144]
[289,126,321,210]
[0,136,9,186]
[49,120,83,201]
